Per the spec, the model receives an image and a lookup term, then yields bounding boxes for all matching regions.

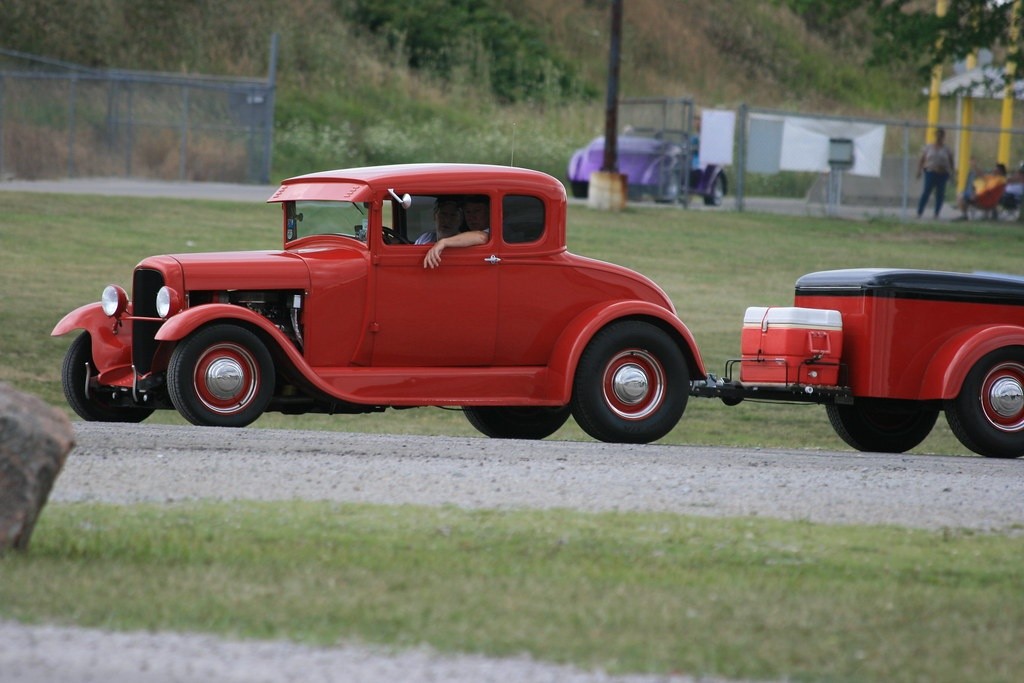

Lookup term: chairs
[968,182,1024,223]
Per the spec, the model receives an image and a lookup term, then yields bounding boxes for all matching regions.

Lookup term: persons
[414,194,490,268]
[914,128,954,220]
[952,154,1024,222]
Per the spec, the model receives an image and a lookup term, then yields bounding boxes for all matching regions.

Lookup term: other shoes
[953,217,967,223]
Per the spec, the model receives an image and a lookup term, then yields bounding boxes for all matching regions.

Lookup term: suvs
[51,162,709,444]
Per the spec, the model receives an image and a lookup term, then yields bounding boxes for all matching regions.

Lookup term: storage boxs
[740,306,843,386]
